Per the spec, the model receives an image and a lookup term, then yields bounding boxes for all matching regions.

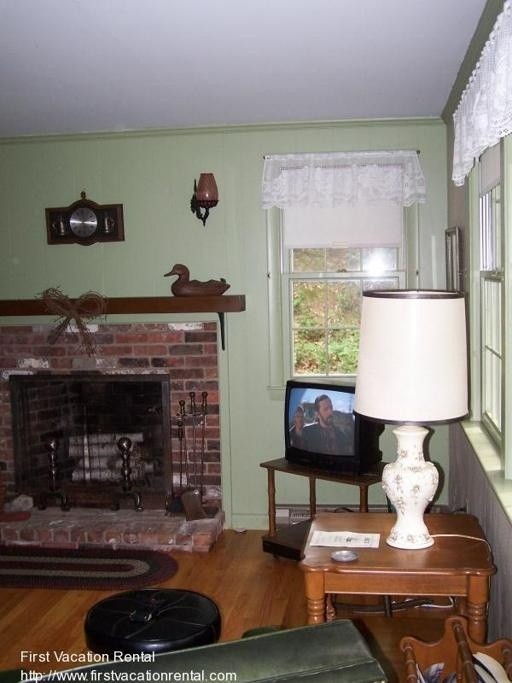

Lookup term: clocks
[45,190,126,247]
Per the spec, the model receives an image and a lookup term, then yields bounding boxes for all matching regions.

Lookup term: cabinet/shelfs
[257,457,393,559]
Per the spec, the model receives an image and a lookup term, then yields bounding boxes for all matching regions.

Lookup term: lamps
[189,172,220,228]
[351,286,472,550]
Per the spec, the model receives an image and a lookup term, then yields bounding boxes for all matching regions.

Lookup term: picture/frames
[444,226,463,293]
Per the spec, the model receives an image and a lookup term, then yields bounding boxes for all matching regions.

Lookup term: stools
[84,585,223,660]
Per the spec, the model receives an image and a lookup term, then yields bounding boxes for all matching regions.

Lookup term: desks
[296,511,499,645]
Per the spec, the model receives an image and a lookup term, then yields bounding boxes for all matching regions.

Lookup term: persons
[287,393,355,458]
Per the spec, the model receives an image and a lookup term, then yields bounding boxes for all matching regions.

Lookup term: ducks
[162,263,232,296]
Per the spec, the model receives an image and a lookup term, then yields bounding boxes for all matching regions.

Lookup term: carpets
[0,546,179,592]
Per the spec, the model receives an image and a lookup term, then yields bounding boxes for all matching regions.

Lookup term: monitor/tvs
[285,378,385,475]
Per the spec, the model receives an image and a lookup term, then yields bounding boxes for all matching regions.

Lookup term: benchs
[0,615,391,682]
